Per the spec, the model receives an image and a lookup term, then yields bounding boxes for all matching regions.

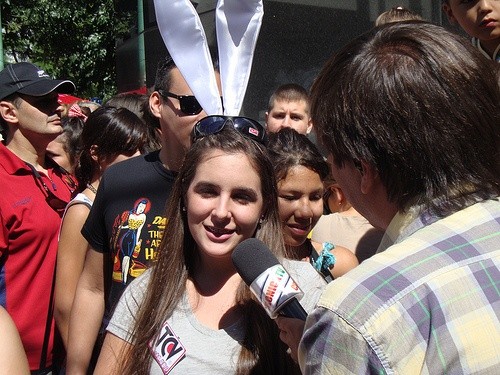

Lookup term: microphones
[230,238,308,322]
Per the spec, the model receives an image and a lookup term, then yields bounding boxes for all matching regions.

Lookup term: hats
[0,62,76,100]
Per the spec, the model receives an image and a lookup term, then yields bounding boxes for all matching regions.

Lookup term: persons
[0,0,500,375]
[310,160,385,266]
[265,82,313,137]
[51,104,146,374]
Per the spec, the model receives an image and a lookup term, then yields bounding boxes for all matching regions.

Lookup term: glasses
[191,115,269,147]
[160,89,225,115]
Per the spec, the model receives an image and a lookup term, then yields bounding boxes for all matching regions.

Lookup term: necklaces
[85,180,98,201]
